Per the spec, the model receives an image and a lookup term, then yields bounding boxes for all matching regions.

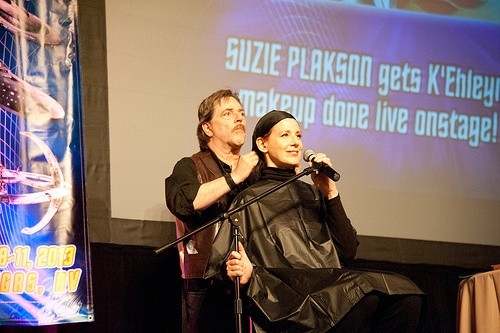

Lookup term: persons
[203,110,425,333]
[164,89,259,333]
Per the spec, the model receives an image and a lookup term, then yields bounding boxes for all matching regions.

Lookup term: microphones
[303,149,340,182]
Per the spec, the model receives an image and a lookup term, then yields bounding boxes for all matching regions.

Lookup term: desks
[457,269,500,333]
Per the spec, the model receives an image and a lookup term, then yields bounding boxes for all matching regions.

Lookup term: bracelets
[224,173,238,191]
